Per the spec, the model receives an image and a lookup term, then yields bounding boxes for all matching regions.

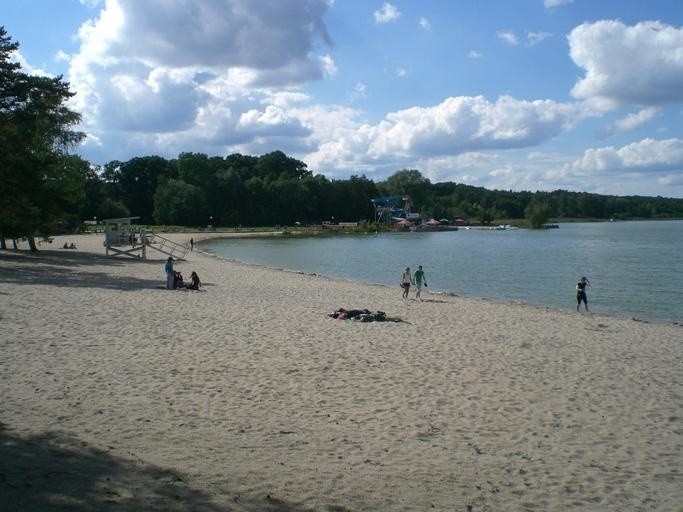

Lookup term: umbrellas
[425,219,440,228]
[394,220,411,228]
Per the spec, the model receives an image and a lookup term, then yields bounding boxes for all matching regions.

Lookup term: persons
[413,265,427,300]
[165,257,174,290]
[63,242,77,249]
[401,267,414,299]
[189,237,193,252]
[576,276,591,313]
[111,226,135,245]
[186,271,200,290]
